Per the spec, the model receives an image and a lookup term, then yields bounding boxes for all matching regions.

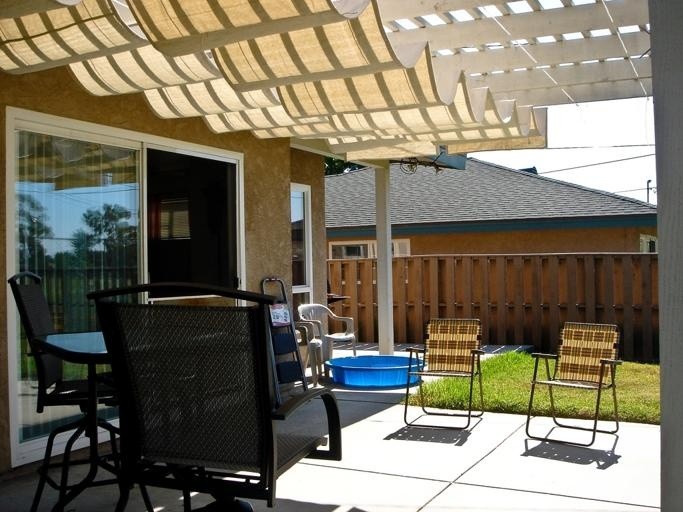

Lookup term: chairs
[403,318,483,430]
[525,321,622,446]
